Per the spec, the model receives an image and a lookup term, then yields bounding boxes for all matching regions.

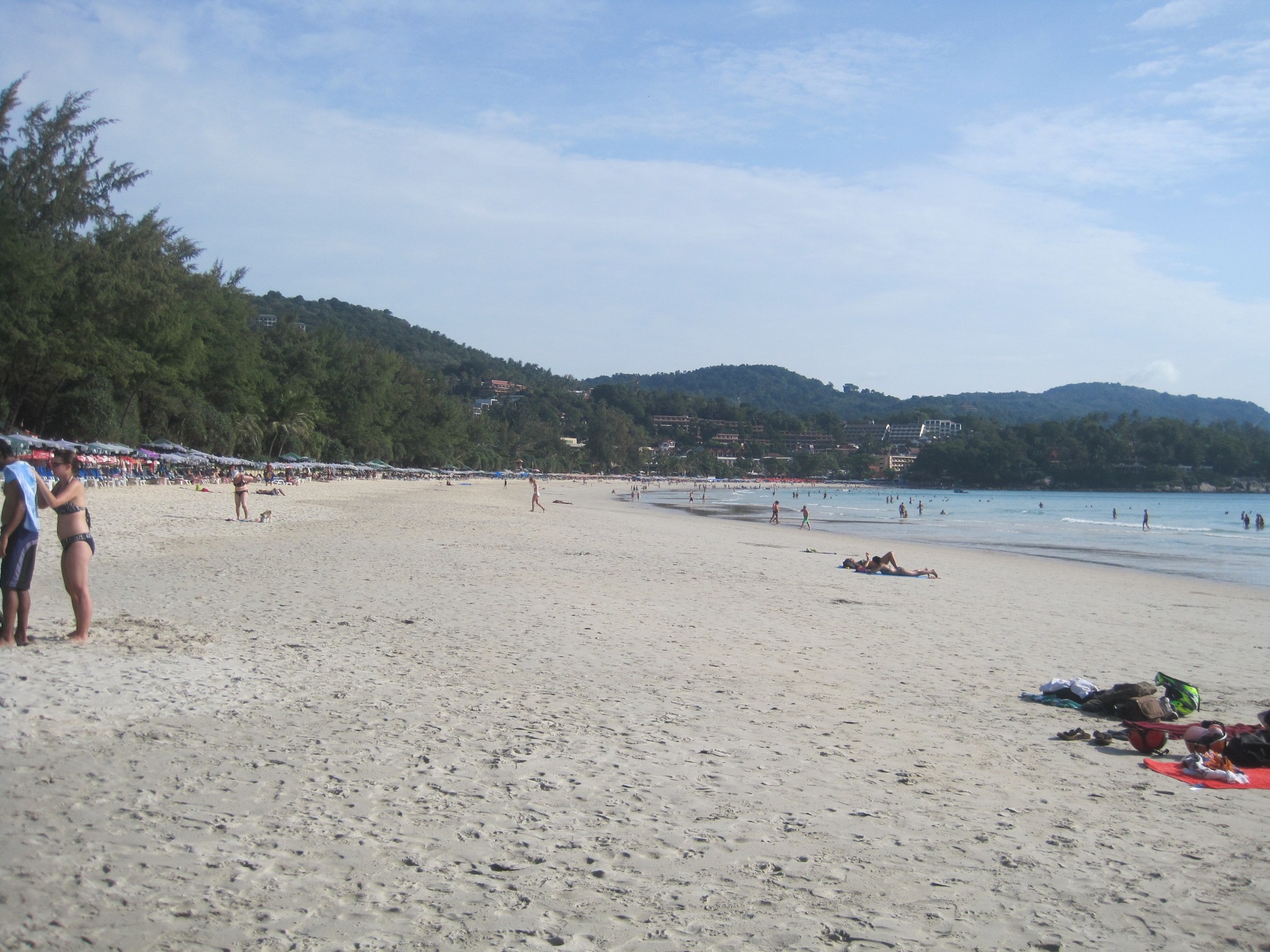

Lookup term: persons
[529,478,545,513]
[358,469,427,481]
[232,470,253,520]
[770,488,827,530]
[1113,508,1117,518]
[1039,502,1043,508]
[0,439,95,646]
[447,481,451,485]
[256,460,378,495]
[1241,510,1264,528]
[1142,509,1150,531]
[503,479,507,489]
[886,495,945,519]
[631,485,647,501]
[689,481,761,504]
[612,490,615,493]
[843,552,938,578]
[159,460,236,483]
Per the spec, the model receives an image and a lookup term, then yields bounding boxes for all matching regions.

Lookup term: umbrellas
[356,458,431,475]
[185,449,358,475]
[429,464,502,477]
[501,469,540,478]
[0,432,188,480]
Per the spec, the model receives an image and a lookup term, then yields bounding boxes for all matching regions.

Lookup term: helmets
[1183,721,1228,755]
[1126,728,1166,754]
[1154,672,1201,716]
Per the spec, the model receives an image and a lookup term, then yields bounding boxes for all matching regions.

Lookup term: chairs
[36,467,222,492]
[269,475,356,486]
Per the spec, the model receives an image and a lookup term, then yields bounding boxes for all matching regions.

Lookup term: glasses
[52,461,68,468]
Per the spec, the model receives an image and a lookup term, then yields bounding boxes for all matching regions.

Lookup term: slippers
[1057,727,1091,741]
[1092,730,1113,745]
